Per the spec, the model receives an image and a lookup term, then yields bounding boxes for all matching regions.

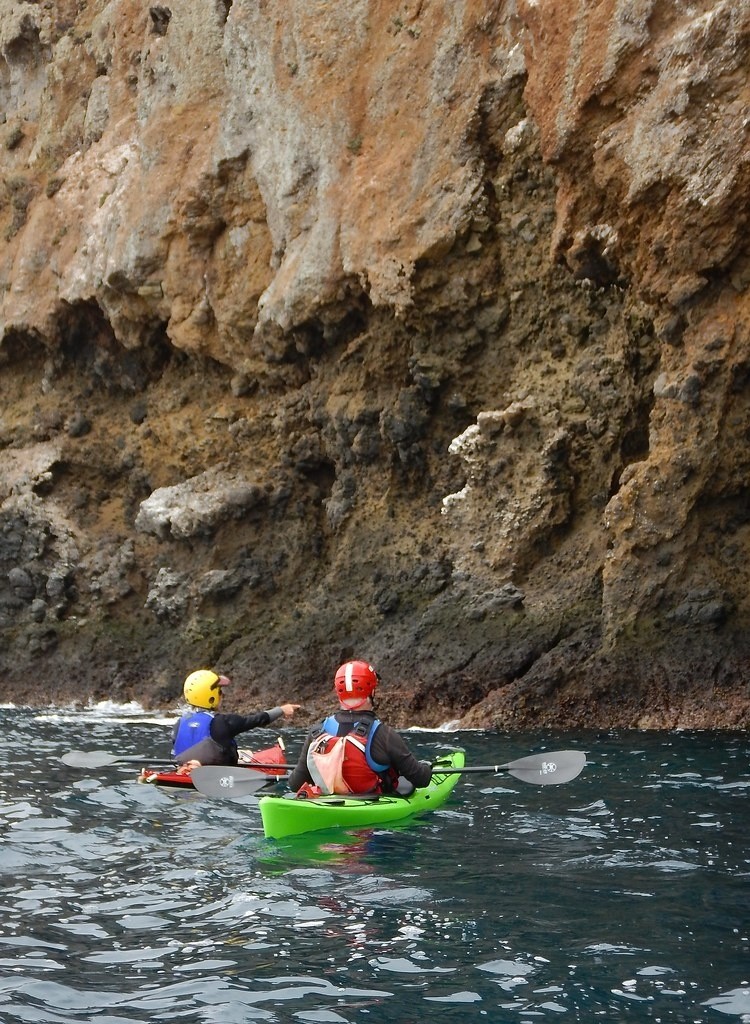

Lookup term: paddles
[63,750,298,768]
[191,749,587,801]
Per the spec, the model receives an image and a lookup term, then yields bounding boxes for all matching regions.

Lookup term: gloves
[420,758,432,770]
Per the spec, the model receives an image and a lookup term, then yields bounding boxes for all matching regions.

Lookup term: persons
[288,661,436,798]
[168,670,301,771]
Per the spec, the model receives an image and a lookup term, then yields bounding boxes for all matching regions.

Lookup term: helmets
[183,669,229,710]
[333,660,379,701]
